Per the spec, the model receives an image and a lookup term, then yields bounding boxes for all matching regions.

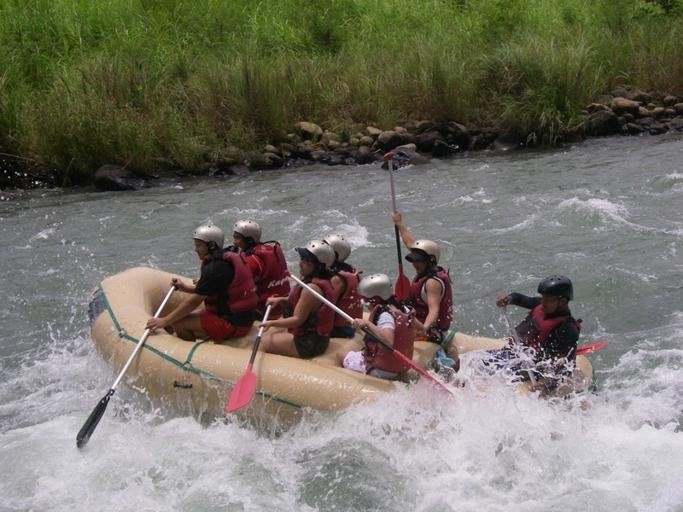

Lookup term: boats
[87,266,595,443]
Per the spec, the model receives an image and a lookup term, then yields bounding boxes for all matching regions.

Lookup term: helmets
[233,219,264,243]
[192,224,225,248]
[323,233,352,263]
[409,238,440,266]
[295,240,335,271]
[538,274,574,303]
[358,273,392,303]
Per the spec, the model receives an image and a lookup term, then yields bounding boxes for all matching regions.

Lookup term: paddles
[574,340,607,356]
[228,305,273,414]
[77,286,177,449]
[284,270,455,394]
[384,152,411,300]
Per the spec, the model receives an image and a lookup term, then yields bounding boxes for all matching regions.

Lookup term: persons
[322,236,364,339]
[481,274,581,397]
[233,217,291,322]
[335,274,414,380]
[254,240,337,358]
[145,223,258,342]
[390,210,452,345]
